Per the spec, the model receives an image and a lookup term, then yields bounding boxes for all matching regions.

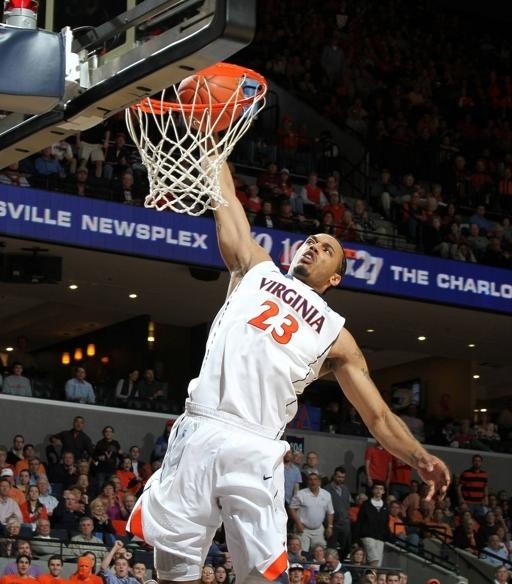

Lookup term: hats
[289,562,304,572]
[0,468,13,478]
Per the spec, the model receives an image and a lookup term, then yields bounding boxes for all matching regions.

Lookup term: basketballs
[179,72,245,133]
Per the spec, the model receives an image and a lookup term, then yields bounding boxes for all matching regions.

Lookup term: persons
[65,367,97,403]
[3,85,213,218]
[1,418,176,583]
[321,402,372,437]
[201,522,236,583]
[125,130,452,584]
[285,441,512,584]
[399,393,512,454]
[227,1,510,269]
[115,369,163,412]
[3,363,32,397]
